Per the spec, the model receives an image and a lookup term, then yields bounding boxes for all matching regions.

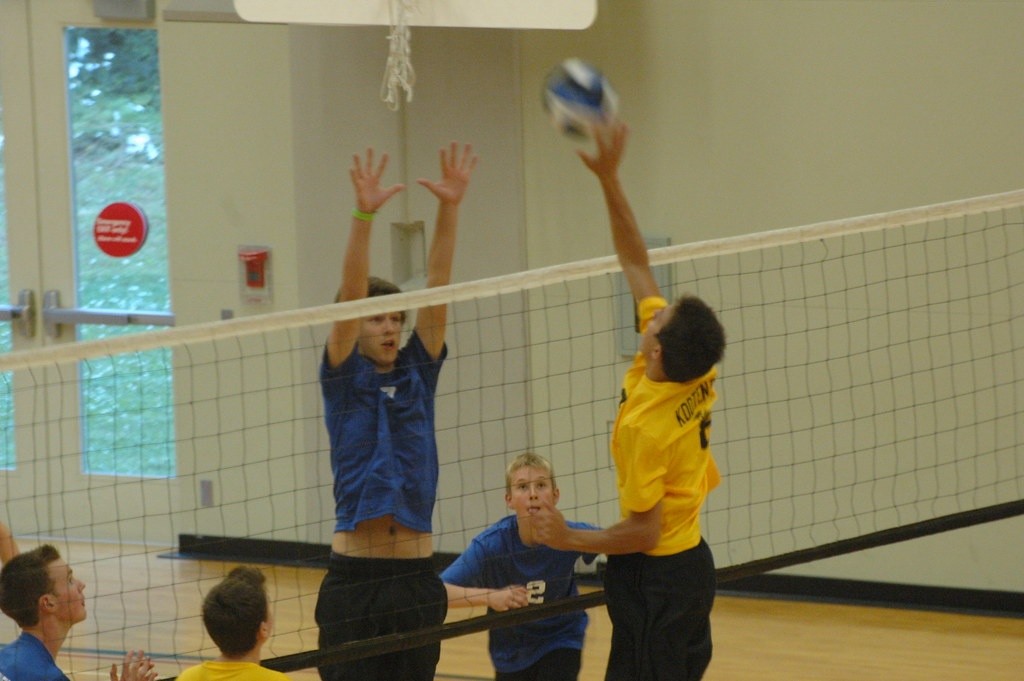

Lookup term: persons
[311,140,476,680]
[0,545,158,681]
[530,123,725,681]
[439,453,608,681]
[175,566,289,681]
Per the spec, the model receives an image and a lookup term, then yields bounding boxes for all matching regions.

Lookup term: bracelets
[352,208,379,223]
